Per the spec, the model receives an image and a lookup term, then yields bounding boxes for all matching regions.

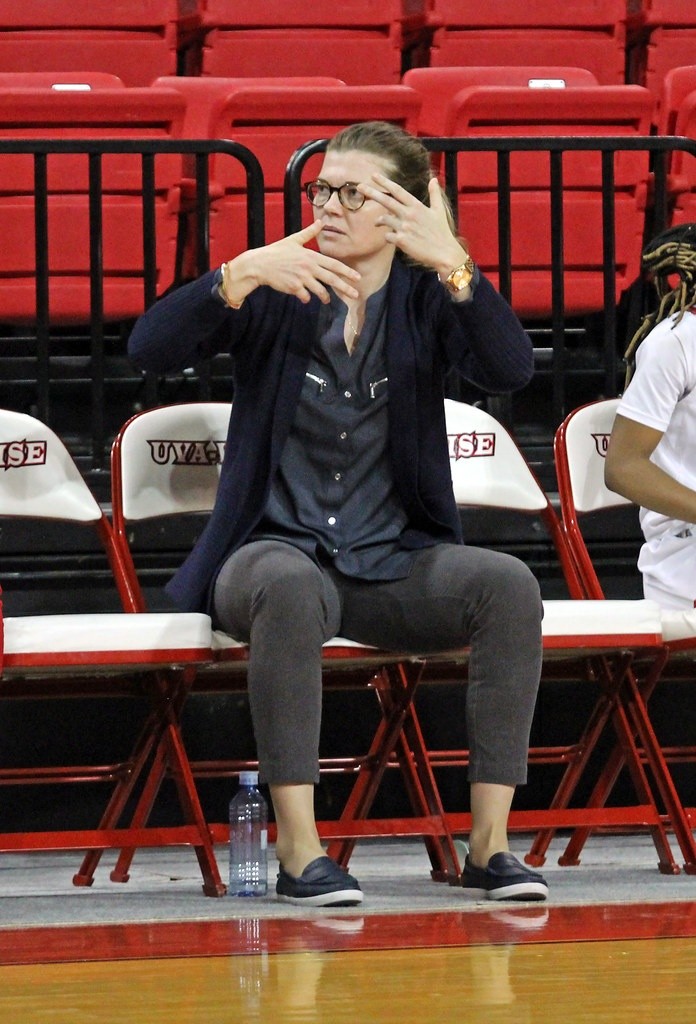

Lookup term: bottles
[227,771,270,896]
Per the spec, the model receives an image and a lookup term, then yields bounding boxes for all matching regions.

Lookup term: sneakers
[275,854,363,908]
[459,852,549,904]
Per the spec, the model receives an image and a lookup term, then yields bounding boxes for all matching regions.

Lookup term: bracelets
[219,261,245,309]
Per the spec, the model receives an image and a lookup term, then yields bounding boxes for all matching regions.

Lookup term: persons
[126,119,556,909]
[602,221,696,616]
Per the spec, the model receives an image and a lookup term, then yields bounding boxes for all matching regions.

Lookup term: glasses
[304,180,392,212]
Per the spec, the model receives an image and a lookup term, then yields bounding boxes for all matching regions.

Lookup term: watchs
[437,254,474,291]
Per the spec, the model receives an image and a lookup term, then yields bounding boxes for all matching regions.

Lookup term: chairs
[562,387,692,874]
[330,391,679,874]
[1,405,227,899]
[89,399,477,901]
